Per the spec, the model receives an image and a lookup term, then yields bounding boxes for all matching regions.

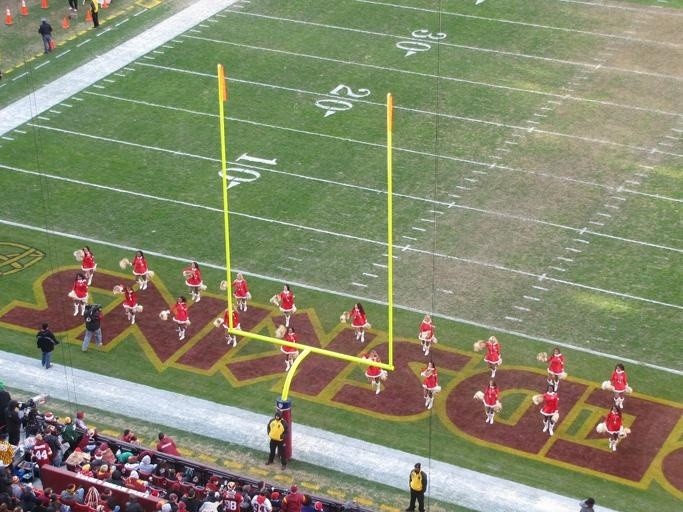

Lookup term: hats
[64,460,353,512]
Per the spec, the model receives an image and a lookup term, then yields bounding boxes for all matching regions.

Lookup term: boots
[73,272,149,325]
[178,292,247,348]
[423,344,497,425]
[542,378,623,452]
[284,315,382,395]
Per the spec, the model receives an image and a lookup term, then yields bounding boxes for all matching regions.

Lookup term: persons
[473,378,503,424]
[596,405,631,451]
[473,336,502,377]
[68,0,112,27]
[602,363,632,409]
[532,385,560,435]
[536,348,567,391]
[38,19,53,54]
[580,497,594,512]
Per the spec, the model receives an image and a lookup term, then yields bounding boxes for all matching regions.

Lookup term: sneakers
[265,460,287,471]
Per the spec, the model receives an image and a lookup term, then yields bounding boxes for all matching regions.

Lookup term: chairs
[23,452,205,512]
